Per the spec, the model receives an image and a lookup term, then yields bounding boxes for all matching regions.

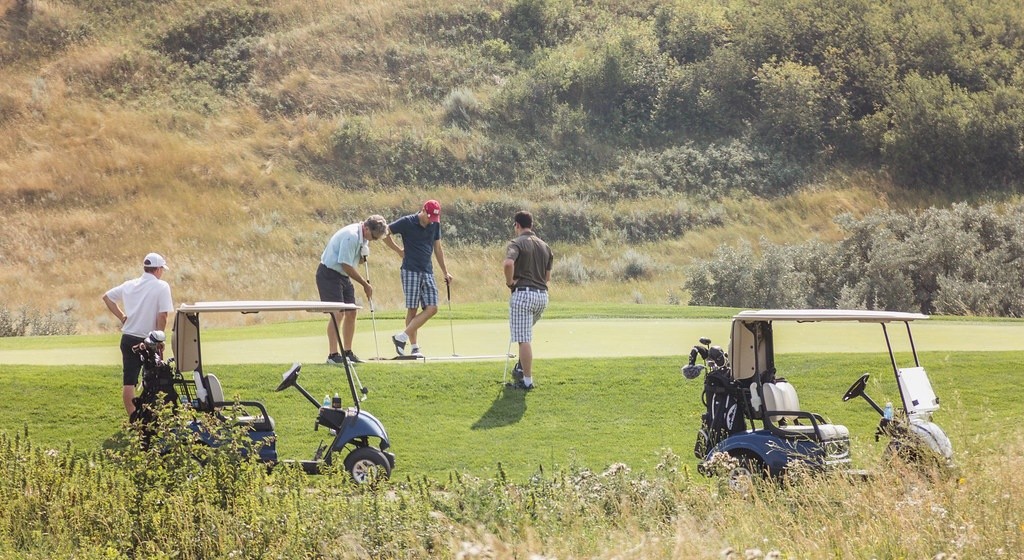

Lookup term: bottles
[885,403,894,419]
[323,395,331,408]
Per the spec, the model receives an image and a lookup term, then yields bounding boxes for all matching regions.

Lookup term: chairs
[204,374,276,431]
[758,380,850,441]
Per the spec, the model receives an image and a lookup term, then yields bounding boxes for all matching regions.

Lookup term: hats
[142,252,170,271]
[423,199,441,224]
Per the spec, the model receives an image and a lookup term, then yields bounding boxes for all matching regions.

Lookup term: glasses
[371,232,379,240]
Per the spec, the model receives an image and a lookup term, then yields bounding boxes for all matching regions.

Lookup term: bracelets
[506,282,514,288]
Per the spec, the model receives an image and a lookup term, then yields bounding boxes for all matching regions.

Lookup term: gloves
[156,341,165,353]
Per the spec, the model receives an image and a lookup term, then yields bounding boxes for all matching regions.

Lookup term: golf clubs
[446,278,458,357]
[363,255,380,361]
[502,337,512,385]
[682,337,727,379]
[130,329,167,367]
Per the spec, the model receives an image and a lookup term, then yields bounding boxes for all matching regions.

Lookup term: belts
[510,287,546,292]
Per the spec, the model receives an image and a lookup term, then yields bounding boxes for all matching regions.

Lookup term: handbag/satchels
[701,365,747,454]
[128,361,181,450]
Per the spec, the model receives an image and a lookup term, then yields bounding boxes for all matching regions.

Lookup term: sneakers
[391,335,406,356]
[410,347,424,358]
[513,380,534,390]
[344,351,364,364]
[327,353,349,366]
[511,363,525,378]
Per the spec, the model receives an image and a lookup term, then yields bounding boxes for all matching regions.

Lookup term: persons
[383,200,453,355]
[504,210,553,389]
[102,252,174,417]
[315,214,389,365]
